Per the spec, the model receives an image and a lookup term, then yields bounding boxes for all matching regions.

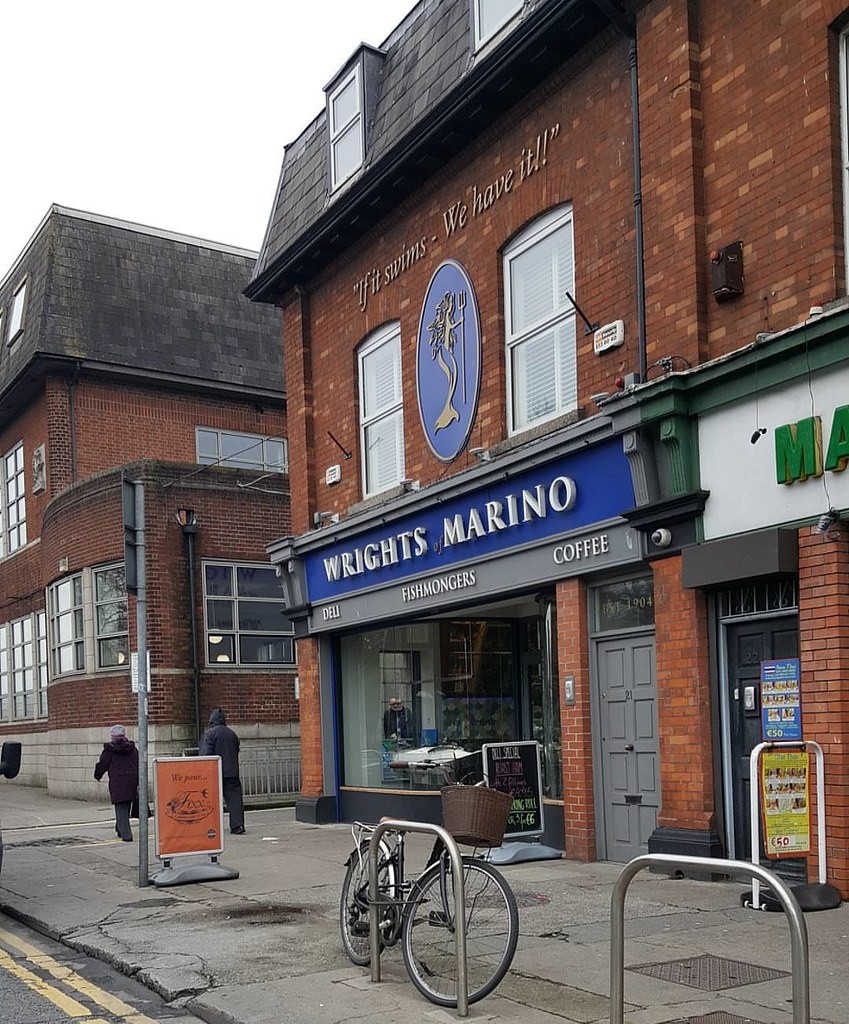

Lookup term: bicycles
[340,759,519,1008]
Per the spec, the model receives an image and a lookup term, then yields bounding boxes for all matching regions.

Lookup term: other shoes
[115,822,121,838]
[231,825,245,834]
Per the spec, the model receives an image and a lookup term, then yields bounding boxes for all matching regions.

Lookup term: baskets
[440,784,515,848]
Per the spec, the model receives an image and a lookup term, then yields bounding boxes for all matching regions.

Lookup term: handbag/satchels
[130,791,151,819]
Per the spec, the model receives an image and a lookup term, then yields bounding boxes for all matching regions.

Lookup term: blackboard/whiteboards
[482,741,545,837]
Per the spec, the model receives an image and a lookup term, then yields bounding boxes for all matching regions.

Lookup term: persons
[198,708,247,834]
[384,697,412,738]
[94,725,139,842]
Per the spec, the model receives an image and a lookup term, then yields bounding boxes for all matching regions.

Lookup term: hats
[111,724,126,738]
